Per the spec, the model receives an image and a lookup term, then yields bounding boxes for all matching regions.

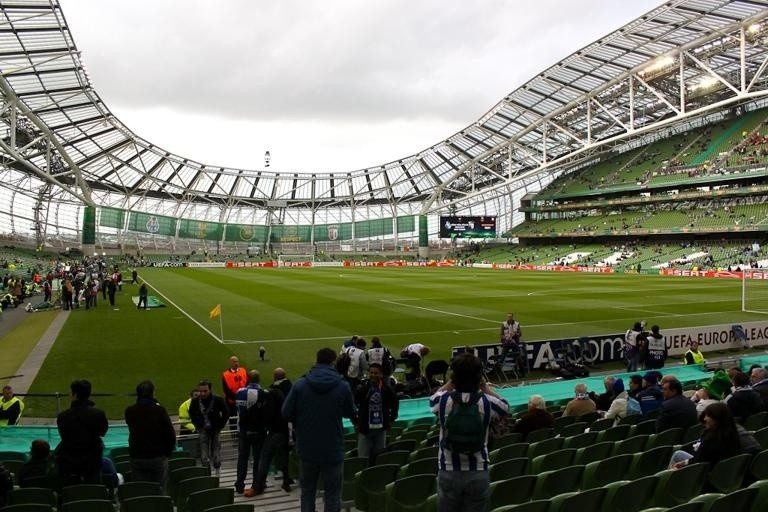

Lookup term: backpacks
[615,397,642,421]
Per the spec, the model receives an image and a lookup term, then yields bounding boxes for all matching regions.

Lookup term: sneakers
[216,467,221,476]
[236,487,262,497]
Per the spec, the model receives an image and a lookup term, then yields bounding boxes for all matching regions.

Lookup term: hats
[643,374,657,383]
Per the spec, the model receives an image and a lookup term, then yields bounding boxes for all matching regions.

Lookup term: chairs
[0,447,257,511]
[265,365,767,512]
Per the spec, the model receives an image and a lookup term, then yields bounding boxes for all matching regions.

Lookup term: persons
[452,115,768,271]
[1,312,767,512]
[1,246,149,312]
[149,250,432,268]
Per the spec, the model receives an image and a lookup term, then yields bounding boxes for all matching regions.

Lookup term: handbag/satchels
[441,393,485,453]
